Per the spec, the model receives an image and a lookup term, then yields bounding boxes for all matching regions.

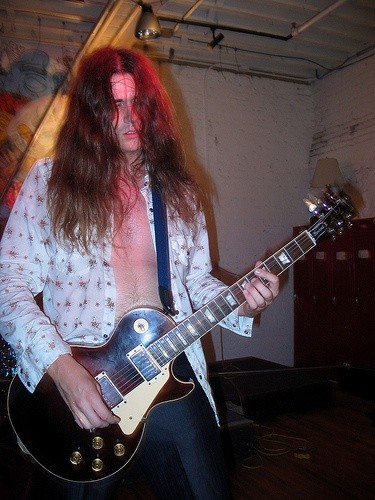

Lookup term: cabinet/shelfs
[290,216,375,406]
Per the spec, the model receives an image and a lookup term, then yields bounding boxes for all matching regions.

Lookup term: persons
[0,46,282,499]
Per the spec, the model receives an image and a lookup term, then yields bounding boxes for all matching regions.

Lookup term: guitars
[5,176,361,485]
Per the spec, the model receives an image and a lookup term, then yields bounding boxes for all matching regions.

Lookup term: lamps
[208,33,225,49]
[136,4,163,41]
